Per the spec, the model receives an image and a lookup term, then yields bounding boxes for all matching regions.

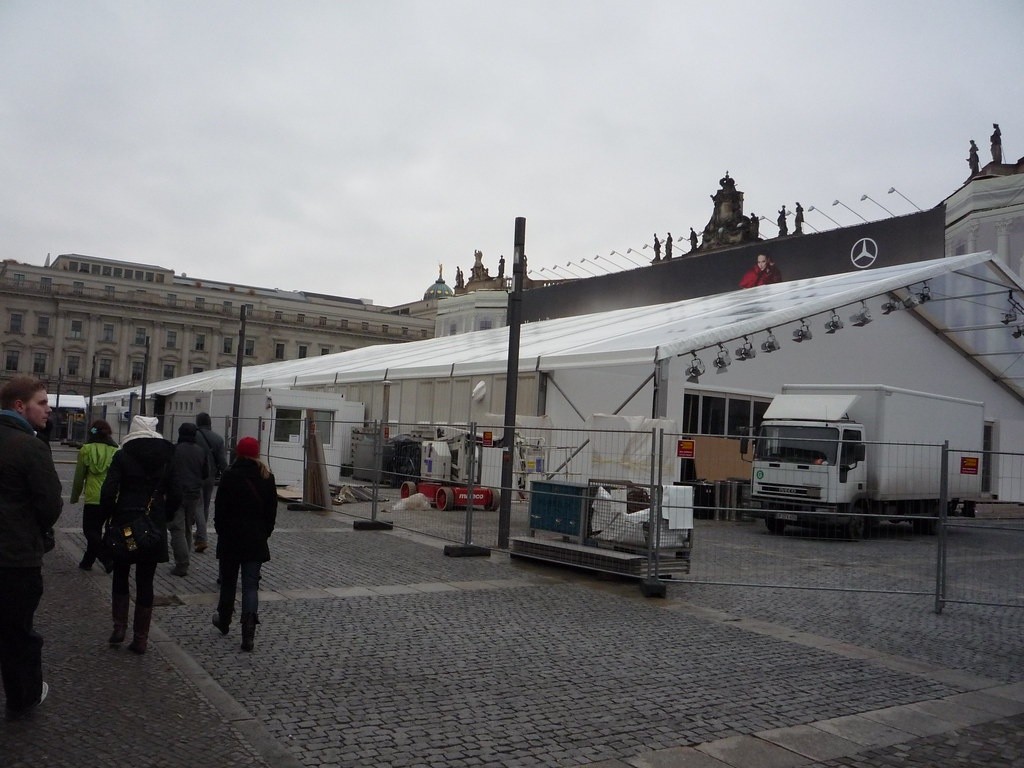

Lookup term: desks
[529,479,625,543]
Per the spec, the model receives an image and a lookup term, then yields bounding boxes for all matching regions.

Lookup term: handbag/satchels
[108,514,164,564]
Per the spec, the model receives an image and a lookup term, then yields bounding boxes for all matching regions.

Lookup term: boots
[128,603,153,653]
[211,581,236,635]
[240,612,262,650]
[108,594,130,645]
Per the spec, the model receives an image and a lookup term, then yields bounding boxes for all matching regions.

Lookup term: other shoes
[104,560,114,574]
[170,570,181,575]
[79,561,91,570]
[8,683,48,719]
[195,543,207,552]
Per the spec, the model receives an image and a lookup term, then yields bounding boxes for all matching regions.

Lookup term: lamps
[686,281,932,383]
[529,187,925,279]
[1000,305,1024,338]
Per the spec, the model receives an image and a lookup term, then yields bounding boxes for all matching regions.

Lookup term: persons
[167,421,210,576]
[777,205,786,226]
[524,254,527,272]
[794,201,805,229]
[654,233,661,251]
[98,415,183,654]
[455,266,460,286]
[70,419,120,576]
[690,227,698,248]
[212,437,278,650]
[966,123,1001,173]
[498,254,506,276]
[665,232,673,254]
[0,374,63,720]
[709,178,737,203]
[739,253,783,289]
[474,249,482,267]
[750,213,759,234]
[192,413,227,553]
[460,271,463,288]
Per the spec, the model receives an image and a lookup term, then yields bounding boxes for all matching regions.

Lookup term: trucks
[740,383,984,542]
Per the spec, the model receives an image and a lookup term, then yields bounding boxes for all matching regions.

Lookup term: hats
[236,437,259,458]
